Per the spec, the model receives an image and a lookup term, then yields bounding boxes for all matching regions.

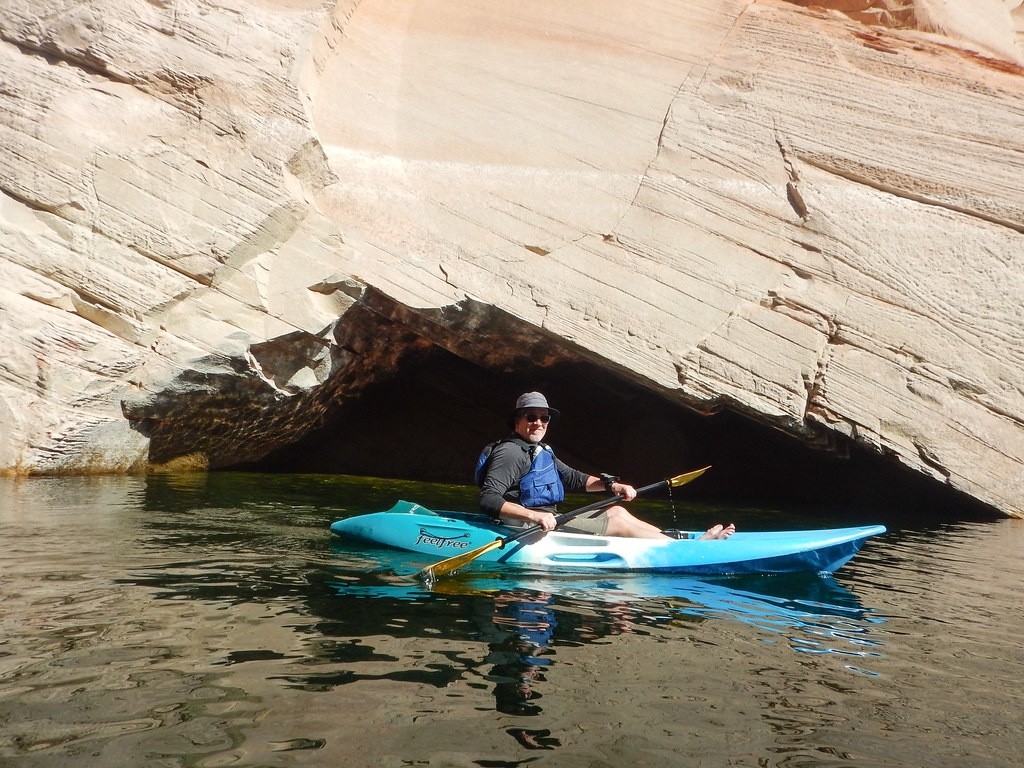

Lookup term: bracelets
[604,480,617,493]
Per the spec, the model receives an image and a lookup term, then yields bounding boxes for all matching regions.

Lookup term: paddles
[419,464,714,581]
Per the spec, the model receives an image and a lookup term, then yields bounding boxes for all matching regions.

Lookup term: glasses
[522,413,552,423]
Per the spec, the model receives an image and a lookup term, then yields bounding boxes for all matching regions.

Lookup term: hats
[512,391,560,416]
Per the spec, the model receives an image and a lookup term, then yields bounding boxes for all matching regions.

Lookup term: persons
[476,391,735,548]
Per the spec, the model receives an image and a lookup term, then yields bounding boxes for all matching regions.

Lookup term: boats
[330,499,887,575]
